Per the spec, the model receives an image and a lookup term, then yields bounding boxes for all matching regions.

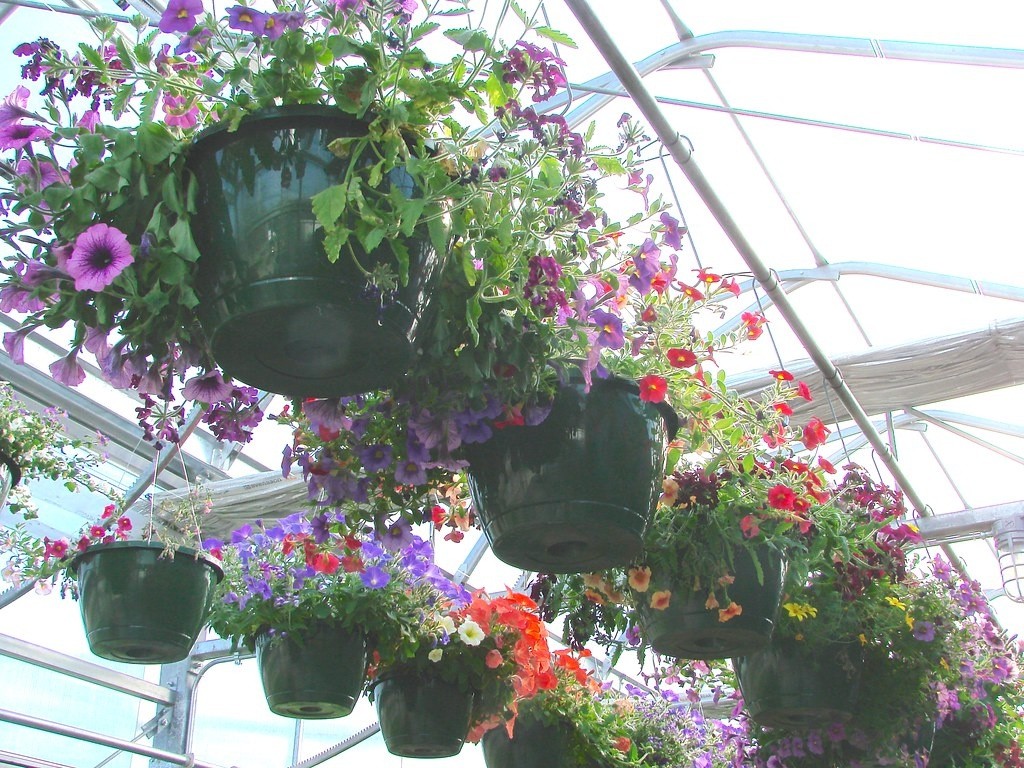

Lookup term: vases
[0,450,21,513]
[480,713,585,767]
[253,618,379,720]
[733,632,861,730]
[72,542,225,665]
[463,370,682,574]
[185,108,452,399]
[628,541,789,661]
[369,669,476,759]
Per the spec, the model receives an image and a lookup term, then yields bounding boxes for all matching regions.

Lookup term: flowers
[0,0,1024,768]
